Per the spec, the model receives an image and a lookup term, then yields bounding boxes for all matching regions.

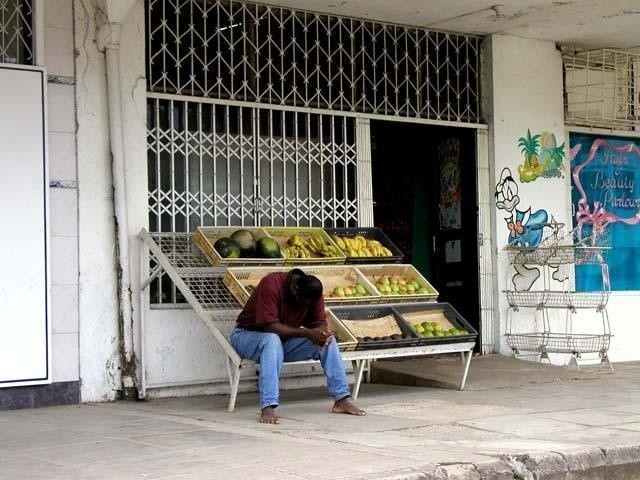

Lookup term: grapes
[524,153,538,168]
[540,169,564,179]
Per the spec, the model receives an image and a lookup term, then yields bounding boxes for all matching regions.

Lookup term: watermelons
[231,230,257,249]
[257,237,280,258]
[214,237,240,258]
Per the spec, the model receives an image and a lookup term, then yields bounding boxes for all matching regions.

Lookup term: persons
[231,268,367,424]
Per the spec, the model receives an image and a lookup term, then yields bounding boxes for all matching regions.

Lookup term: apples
[376,274,428,294]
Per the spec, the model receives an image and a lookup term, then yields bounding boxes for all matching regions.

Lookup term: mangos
[334,284,371,296]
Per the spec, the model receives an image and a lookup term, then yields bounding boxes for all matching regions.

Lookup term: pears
[414,322,470,337]
[522,170,534,182]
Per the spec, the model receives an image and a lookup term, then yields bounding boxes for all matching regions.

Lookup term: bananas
[283,232,393,258]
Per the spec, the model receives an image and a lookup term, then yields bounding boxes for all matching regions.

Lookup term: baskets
[192,226,479,351]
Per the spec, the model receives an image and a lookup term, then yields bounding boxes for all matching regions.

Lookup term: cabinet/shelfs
[502,245,614,373]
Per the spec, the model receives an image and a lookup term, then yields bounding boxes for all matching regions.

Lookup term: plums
[356,333,412,342]
